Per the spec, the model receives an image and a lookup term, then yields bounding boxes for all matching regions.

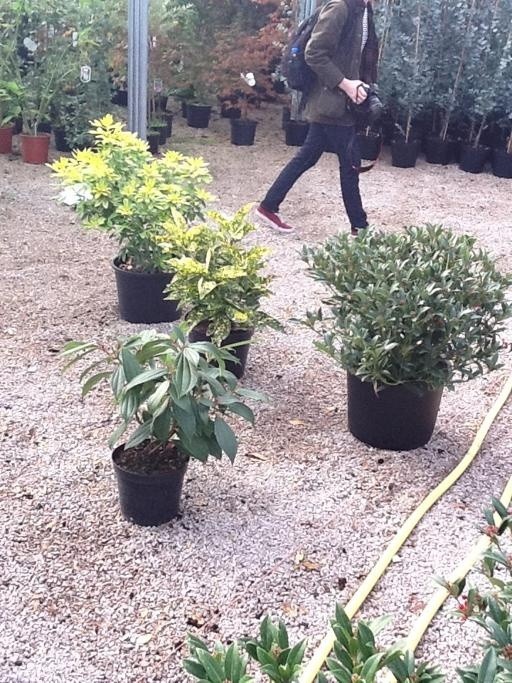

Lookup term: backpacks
[282,0,355,89]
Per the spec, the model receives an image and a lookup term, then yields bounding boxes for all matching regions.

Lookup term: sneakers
[349,227,358,236]
[254,205,297,233]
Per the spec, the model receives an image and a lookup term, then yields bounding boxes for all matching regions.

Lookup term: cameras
[347,82,383,125]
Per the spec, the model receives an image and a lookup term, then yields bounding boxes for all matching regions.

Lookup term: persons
[253,0,379,237]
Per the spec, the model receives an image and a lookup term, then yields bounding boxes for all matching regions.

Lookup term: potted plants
[45,111,220,324]
[287,220,512,452]
[1,0,512,179]
[156,203,286,382]
[52,324,266,526]
